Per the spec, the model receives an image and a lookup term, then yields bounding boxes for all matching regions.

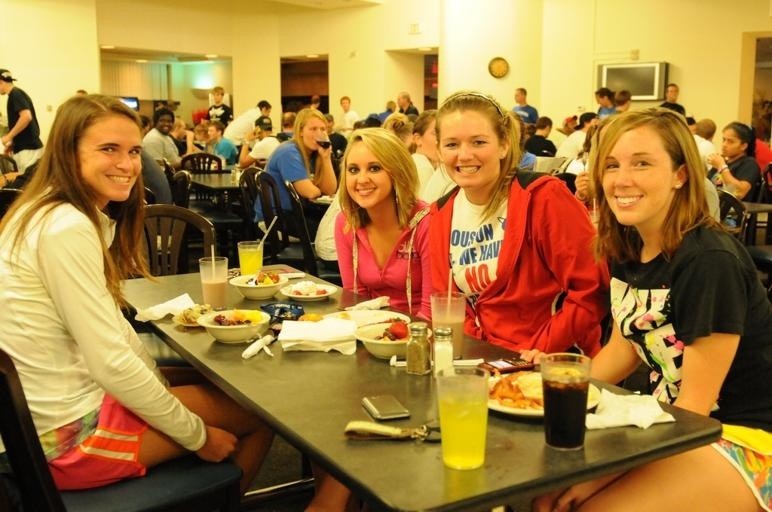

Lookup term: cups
[199,257,229,310]
[430,291,465,361]
[238,241,264,276]
[436,367,490,470]
[541,351,592,454]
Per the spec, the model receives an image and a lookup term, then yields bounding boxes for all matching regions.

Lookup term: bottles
[406,322,432,375]
[231,170,236,184]
[433,327,454,378]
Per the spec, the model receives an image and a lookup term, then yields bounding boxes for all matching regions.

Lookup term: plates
[280,284,337,302]
[324,310,411,324]
[489,370,602,417]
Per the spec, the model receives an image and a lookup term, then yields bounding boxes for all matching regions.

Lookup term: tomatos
[293,291,301,295]
[390,321,407,338]
[316,289,326,295]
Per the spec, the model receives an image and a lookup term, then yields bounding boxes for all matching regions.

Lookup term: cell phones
[479,357,534,375]
[361,394,410,420]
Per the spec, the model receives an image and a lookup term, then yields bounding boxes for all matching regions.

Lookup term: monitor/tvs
[117,97,139,111]
[602,62,666,101]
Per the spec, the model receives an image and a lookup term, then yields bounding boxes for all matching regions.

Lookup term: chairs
[703,159,771,306]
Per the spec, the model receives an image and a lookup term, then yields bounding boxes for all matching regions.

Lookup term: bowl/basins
[200,309,271,345]
[354,321,432,360]
[229,274,288,300]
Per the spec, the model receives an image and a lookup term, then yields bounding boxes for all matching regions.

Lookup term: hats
[574,113,594,130]
[562,116,577,127]
[255,116,272,131]
[0,70,16,81]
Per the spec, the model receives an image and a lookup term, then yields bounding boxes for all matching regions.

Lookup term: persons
[0,64,771,270]
[302,125,438,511]
[415,89,606,368]
[0,89,278,499]
[527,106,772,511]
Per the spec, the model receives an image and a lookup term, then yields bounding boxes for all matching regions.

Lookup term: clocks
[489,56,510,79]
